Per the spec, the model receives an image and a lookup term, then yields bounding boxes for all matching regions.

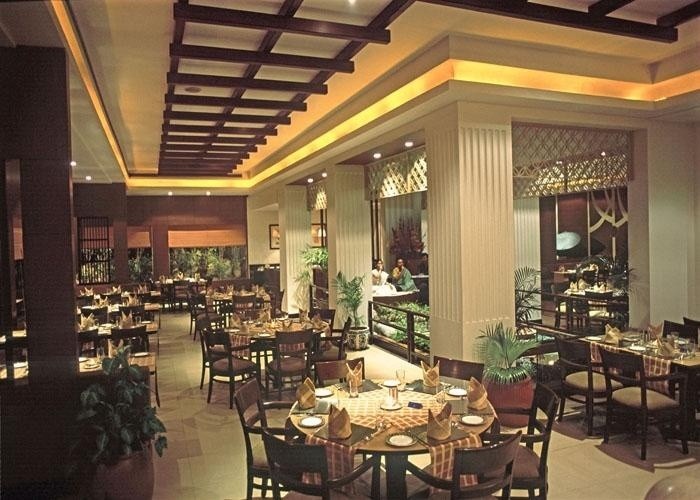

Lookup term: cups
[246,316,251,321]
[349,380,358,397]
[376,411,385,432]
[389,388,398,401]
[672,332,679,349]
[688,339,695,359]
[433,395,442,412]
[435,379,445,395]
[135,316,141,325]
[97,348,104,362]
[396,369,406,392]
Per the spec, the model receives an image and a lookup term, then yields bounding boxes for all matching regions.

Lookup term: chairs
[264,329,313,409]
[187,292,205,334]
[310,307,334,345]
[683,317,699,331]
[312,317,352,382]
[201,329,258,410]
[129,292,151,302]
[233,378,300,500]
[172,280,190,311]
[315,357,366,463]
[100,293,120,304]
[433,355,485,387]
[261,427,380,500]
[77,295,91,307]
[662,320,698,346]
[191,294,223,342]
[119,305,145,321]
[82,307,108,323]
[598,345,689,460]
[401,430,522,500]
[585,291,612,335]
[197,315,241,389]
[554,335,624,437]
[245,309,262,319]
[111,325,147,355]
[480,382,559,500]
[232,295,255,321]
[77,328,98,357]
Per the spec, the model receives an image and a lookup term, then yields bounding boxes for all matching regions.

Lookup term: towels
[137,282,148,295]
[195,272,201,283]
[257,308,271,323]
[158,273,166,284]
[281,318,291,333]
[326,403,352,442]
[225,283,235,295]
[601,324,622,347]
[112,285,122,296]
[295,376,319,411]
[343,361,363,388]
[418,360,442,389]
[423,402,452,443]
[98,295,108,307]
[107,338,124,359]
[176,271,184,281]
[228,312,241,328]
[465,377,490,411]
[75,322,88,332]
[311,313,322,329]
[82,285,94,297]
[298,309,308,323]
[119,309,133,327]
[236,320,252,334]
[79,312,96,327]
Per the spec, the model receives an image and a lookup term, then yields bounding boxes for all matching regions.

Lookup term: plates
[380,402,402,411]
[84,365,99,369]
[629,346,645,353]
[229,329,240,332]
[385,432,417,447]
[383,379,400,387]
[458,413,487,426]
[135,352,148,357]
[79,357,88,362]
[14,362,26,368]
[315,388,333,397]
[586,336,602,341]
[448,388,467,396]
[298,415,325,427]
[142,320,151,324]
[260,333,271,337]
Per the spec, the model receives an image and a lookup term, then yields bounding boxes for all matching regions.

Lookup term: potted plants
[472,321,540,428]
[331,272,371,351]
[76,341,167,500]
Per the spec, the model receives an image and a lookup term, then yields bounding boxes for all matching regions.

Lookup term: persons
[413,253,429,306]
[389,259,416,291]
[371,259,389,294]
